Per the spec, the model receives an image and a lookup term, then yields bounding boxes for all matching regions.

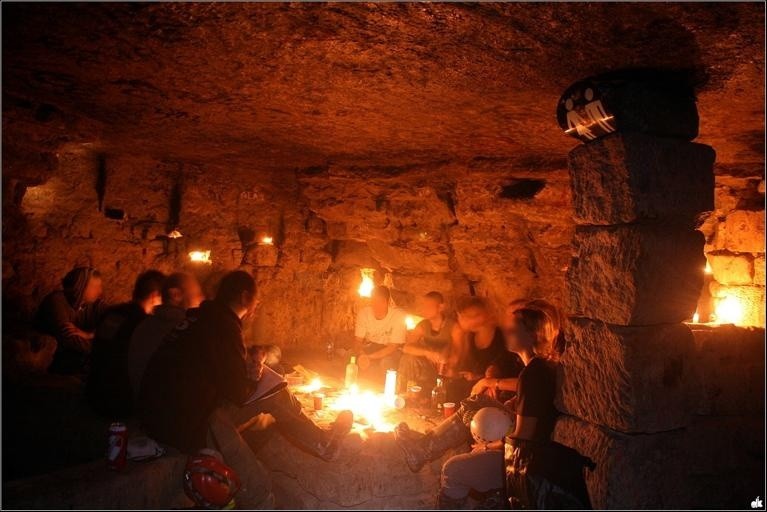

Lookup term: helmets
[470,408,511,444]
[183,455,241,509]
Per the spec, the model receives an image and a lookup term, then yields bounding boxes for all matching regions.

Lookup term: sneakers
[396,424,426,472]
[315,410,352,462]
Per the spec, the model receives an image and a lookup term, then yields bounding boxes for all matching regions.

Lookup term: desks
[2,414,186,510]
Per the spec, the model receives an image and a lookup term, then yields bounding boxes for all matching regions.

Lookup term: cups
[394,397,405,409]
[410,385,421,402]
[313,393,323,410]
[442,402,455,418]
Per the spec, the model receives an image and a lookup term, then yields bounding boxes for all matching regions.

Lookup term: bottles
[344,355,358,389]
[327,343,332,361]
[431,377,446,410]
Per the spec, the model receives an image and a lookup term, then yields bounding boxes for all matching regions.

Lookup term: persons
[398,290,460,388]
[83,268,165,421]
[398,298,561,509]
[343,284,406,378]
[28,266,102,375]
[126,272,205,412]
[138,270,354,464]
